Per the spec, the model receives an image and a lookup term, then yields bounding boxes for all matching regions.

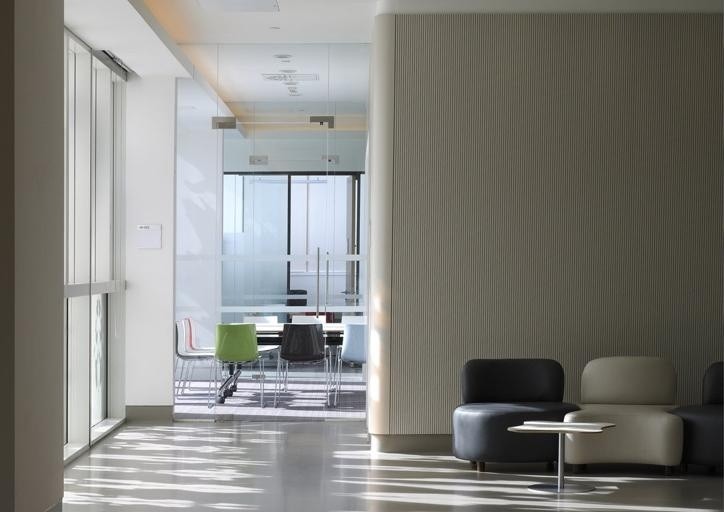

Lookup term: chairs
[175,314,367,408]
[451,354,724,478]
